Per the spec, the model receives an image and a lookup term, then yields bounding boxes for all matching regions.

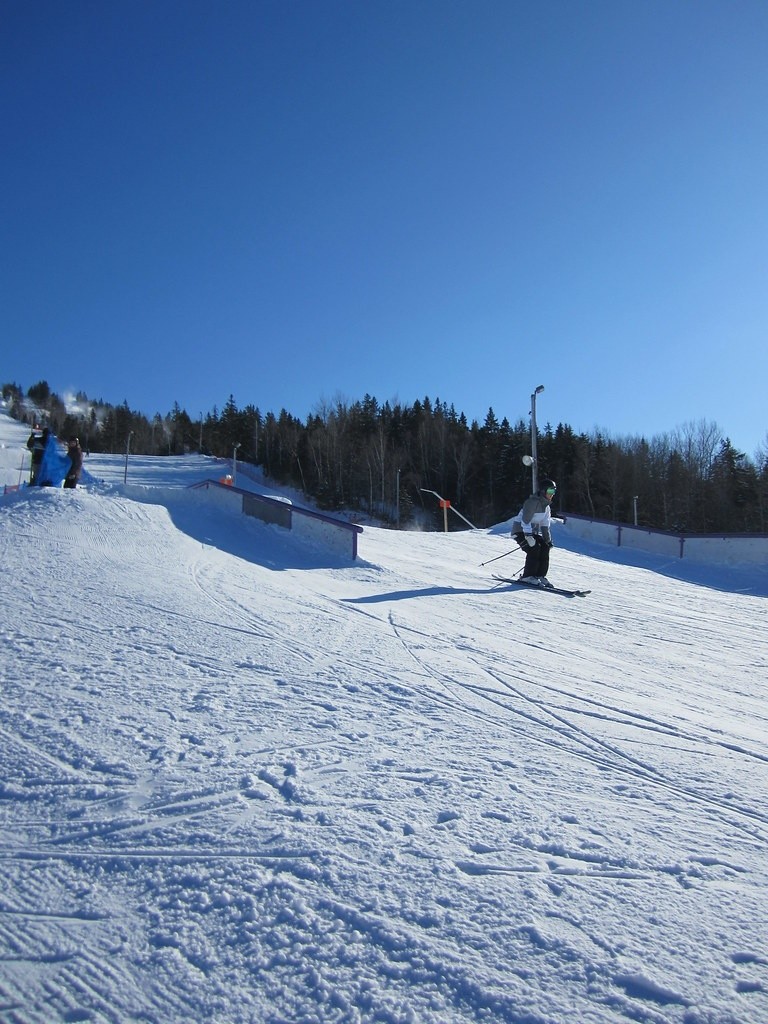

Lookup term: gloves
[525,535,537,547]
[69,474,75,480]
[547,542,554,548]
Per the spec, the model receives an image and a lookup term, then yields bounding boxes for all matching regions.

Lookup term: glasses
[546,486,556,495]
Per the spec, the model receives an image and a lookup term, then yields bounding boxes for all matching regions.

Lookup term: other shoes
[535,575,554,589]
[521,573,545,587]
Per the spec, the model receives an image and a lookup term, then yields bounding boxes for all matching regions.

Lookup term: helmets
[69,437,79,447]
[539,480,556,490]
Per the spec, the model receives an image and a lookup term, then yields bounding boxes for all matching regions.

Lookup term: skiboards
[491,573,591,595]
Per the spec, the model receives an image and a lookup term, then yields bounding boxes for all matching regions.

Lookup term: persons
[85,449,89,458]
[28,428,50,485]
[511,479,566,588]
[59,438,83,488]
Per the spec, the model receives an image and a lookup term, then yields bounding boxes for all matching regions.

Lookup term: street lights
[521,384,545,495]
[199,411,203,454]
[123,430,135,484]
